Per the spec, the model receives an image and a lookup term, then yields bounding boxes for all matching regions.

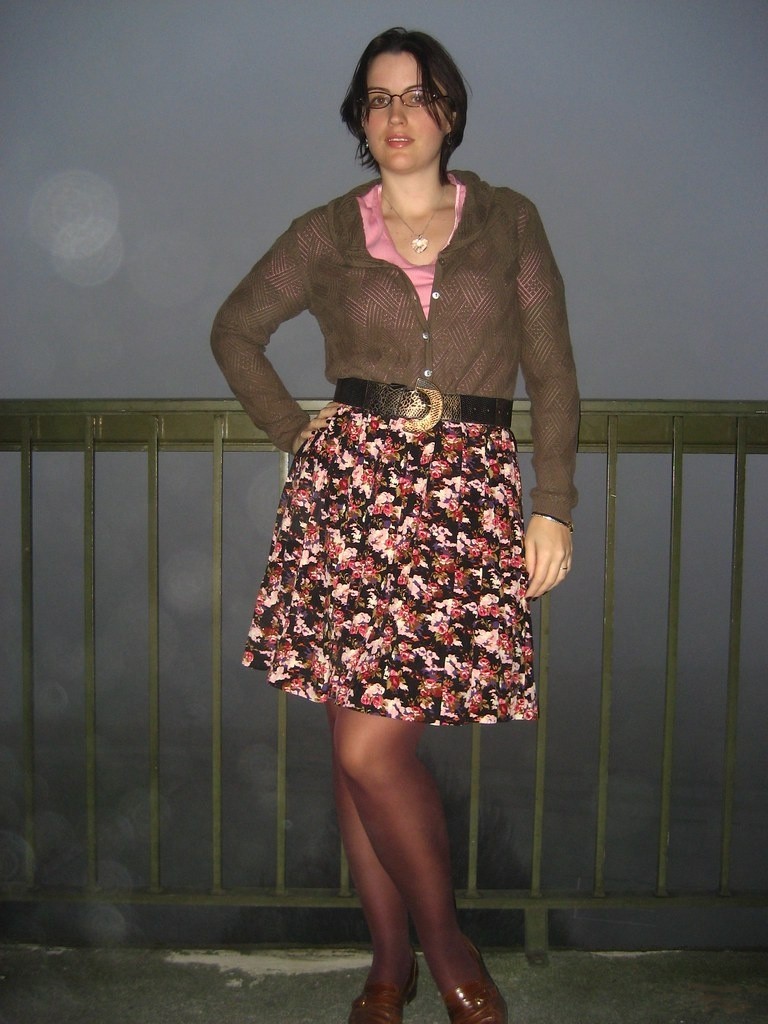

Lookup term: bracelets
[531,512,574,534]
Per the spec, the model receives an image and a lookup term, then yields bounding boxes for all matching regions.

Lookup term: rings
[560,568,567,571]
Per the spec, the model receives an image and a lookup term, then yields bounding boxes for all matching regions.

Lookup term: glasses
[355,90,450,109]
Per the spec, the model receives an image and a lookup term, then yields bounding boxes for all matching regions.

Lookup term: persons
[210,27,579,1024]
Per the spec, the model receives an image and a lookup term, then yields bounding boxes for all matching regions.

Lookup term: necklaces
[382,184,445,254]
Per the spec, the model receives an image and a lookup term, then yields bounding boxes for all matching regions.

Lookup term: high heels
[346,947,417,1024]
[433,933,508,1024]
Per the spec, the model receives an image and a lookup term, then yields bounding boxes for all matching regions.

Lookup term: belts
[333,375,516,432]
